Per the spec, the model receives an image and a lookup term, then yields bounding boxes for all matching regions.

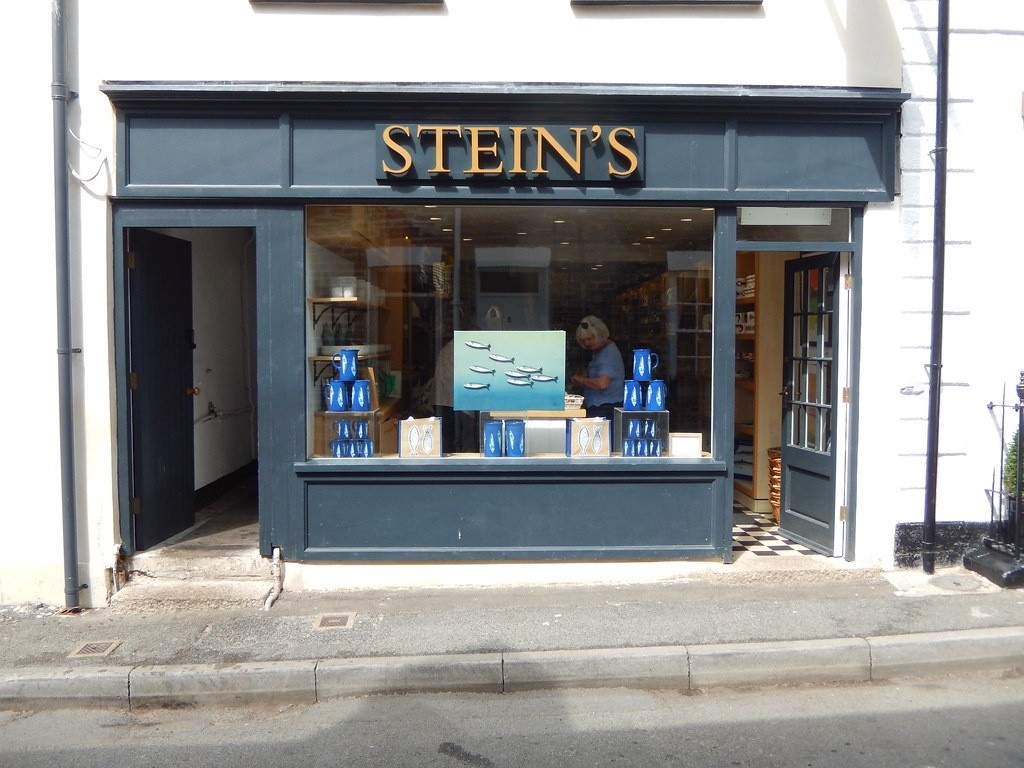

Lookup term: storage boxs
[614,408,669,457]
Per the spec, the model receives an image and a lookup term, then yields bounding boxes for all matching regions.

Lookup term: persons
[433,338,476,453]
[570,316,625,420]
[567,342,587,395]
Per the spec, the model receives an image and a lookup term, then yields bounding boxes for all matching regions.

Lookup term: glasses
[577,322,594,329]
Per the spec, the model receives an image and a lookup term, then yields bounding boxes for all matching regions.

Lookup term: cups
[330,419,374,458]
[484,420,503,457]
[623,419,661,457]
[504,418,525,457]
[735,311,756,335]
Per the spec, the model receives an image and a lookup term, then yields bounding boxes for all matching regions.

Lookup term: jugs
[323,380,348,411]
[632,349,658,381]
[348,379,371,411]
[645,380,667,411]
[623,380,643,411]
[332,349,360,381]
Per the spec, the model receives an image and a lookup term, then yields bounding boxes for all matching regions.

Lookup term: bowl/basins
[328,276,385,305]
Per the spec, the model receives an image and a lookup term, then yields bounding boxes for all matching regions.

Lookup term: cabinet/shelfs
[306,296,395,385]
[314,398,403,455]
[697,249,802,513]
